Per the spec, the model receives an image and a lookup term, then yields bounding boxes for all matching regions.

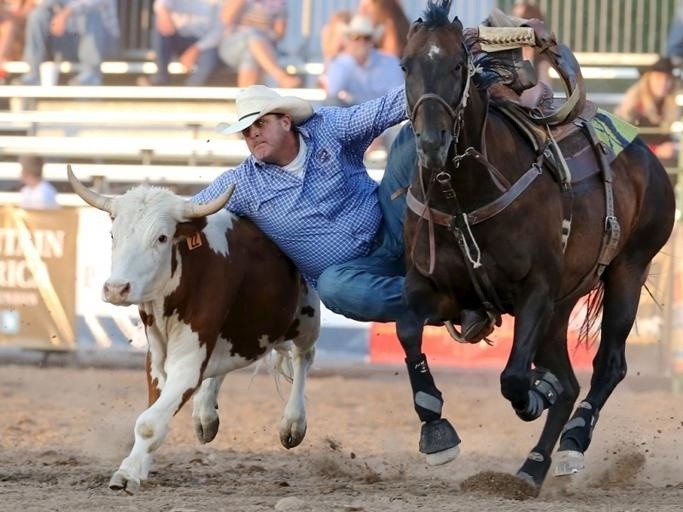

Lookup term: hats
[635,57,674,79]
[335,14,383,44]
[220,83,314,135]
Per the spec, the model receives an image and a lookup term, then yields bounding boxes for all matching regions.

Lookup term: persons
[0,1,405,154]
[17,155,59,210]
[186,84,496,343]
[611,16,683,222]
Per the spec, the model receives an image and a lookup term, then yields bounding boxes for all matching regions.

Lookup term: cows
[64,159,323,498]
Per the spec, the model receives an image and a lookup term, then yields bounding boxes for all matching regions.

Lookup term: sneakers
[459,307,495,344]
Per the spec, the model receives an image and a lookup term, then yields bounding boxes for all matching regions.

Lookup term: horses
[393,1,678,497]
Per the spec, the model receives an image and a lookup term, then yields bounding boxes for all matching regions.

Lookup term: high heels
[500,46,538,95]
